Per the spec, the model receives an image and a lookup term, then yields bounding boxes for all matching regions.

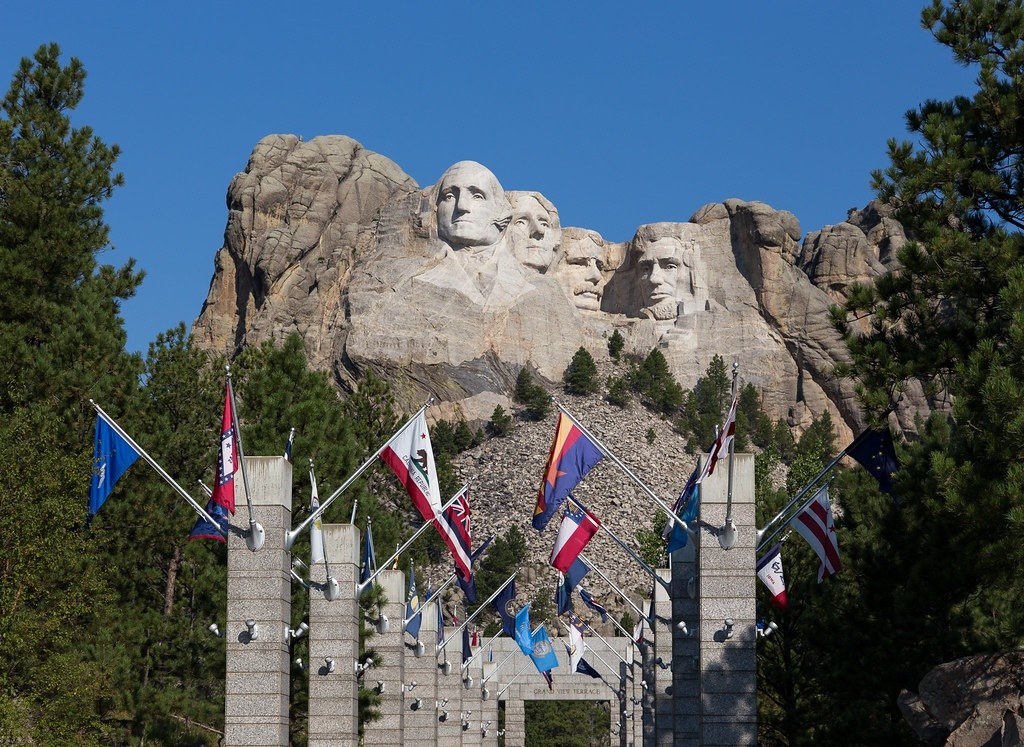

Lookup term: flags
[359,530,372,593]
[791,488,842,584]
[187,384,241,543]
[563,613,601,679]
[662,468,699,554]
[576,584,607,623]
[392,485,560,691]
[532,411,605,531]
[847,415,901,494]
[87,414,139,516]
[378,410,443,526]
[690,396,734,485]
[757,553,786,608]
[551,557,591,617]
[310,474,325,565]
[547,497,600,574]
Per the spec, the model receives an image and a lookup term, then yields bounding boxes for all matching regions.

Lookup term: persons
[500,189,562,275]
[559,226,608,311]
[414,160,536,306]
[636,238,693,320]
